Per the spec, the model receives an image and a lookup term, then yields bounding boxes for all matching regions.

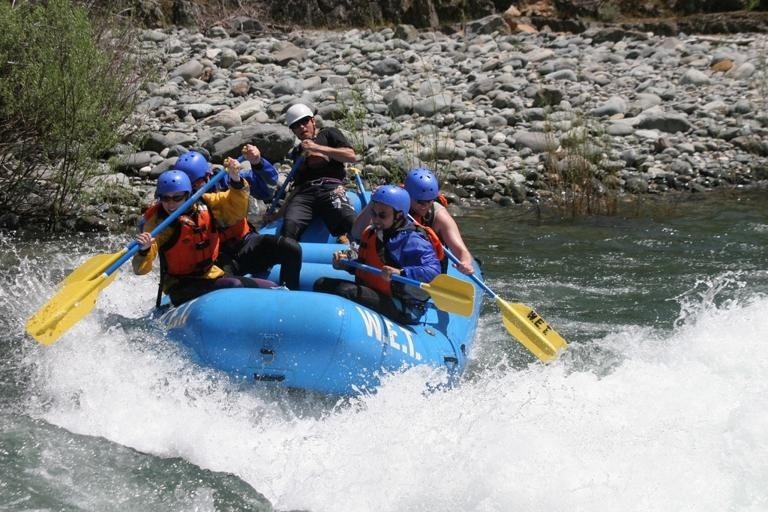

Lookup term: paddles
[338,257,474,317]
[25,166,230,347]
[407,214,568,364]
[57,155,246,299]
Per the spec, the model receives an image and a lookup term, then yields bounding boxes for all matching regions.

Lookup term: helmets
[173,151,214,185]
[404,168,439,202]
[153,169,192,203]
[285,103,315,127]
[370,187,411,233]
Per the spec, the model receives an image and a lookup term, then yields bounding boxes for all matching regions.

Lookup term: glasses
[290,117,313,129]
[414,199,434,205]
[158,193,188,204]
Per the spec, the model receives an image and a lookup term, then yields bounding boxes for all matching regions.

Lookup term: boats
[152,192,483,398]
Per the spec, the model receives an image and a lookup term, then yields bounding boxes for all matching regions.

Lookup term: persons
[351,168,474,275]
[130,156,279,306]
[263,104,356,237]
[313,184,441,325]
[176,144,303,289]
